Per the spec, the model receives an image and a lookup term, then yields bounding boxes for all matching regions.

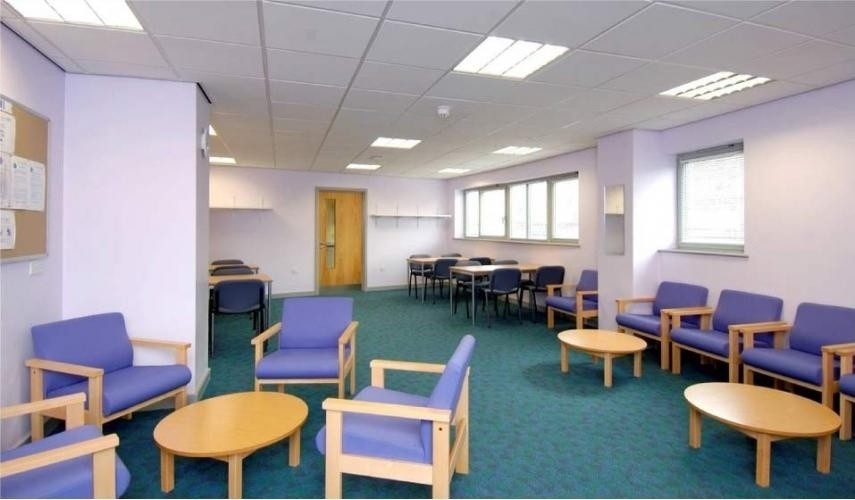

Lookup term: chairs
[835,346,855,440]
[740,301,855,406]
[519,265,564,322]
[24,311,190,435]
[249,294,359,401]
[670,288,784,385]
[454,260,499,320]
[424,258,458,306]
[544,270,599,332]
[408,254,432,298]
[213,265,253,276]
[491,260,536,289]
[482,268,522,328]
[468,257,492,266]
[323,334,476,500]
[211,259,243,265]
[206,278,266,359]
[613,280,712,370]
[0,392,130,499]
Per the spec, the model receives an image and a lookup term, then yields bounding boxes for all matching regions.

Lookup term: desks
[406,257,468,301]
[209,265,259,274]
[449,264,539,327]
[205,272,273,330]
